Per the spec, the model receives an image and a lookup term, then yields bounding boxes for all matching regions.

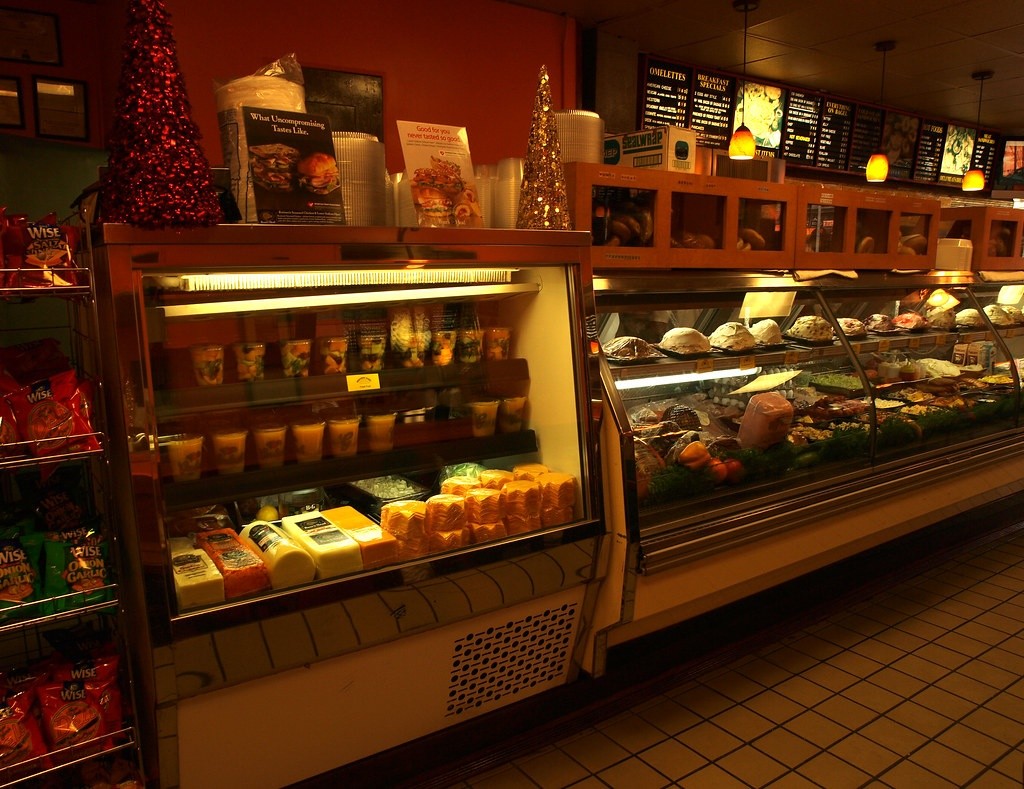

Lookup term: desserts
[602,304,1024,359]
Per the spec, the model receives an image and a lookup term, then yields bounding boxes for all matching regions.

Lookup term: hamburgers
[416,186,454,217]
[296,151,341,195]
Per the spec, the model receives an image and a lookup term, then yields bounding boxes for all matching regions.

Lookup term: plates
[606,321,1024,419]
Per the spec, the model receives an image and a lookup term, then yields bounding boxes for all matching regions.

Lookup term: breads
[858,236,928,256]
[594,205,766,253]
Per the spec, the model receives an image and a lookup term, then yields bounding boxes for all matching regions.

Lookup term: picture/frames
[0,6,62,67]
[29,75,91,143]
[0,75,26,130]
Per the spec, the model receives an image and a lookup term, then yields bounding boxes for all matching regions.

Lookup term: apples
[703,459,743,483]
[255,505,280,521]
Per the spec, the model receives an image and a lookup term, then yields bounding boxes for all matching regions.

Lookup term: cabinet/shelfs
[562,161,798,271]
[132,262,1023,787]
[794,180,941,273]
[0,255,146,789]
[940,205,1024,271]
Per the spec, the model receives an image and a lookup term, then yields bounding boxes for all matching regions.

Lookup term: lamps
[865,39,896,182]
[729,1,760,159]
[962,67,992,192]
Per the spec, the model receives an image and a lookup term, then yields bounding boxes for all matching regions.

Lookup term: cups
[457,324,486,363]
[231,342,266,380]
[911,367,920,380]
[878,364,891,382]
[169,409,398,484]
[901,368,916,381]
[468,396,499,437]
[280,338,312,377]
[356,331,386,370]
[887,367,901,382]
[190,344,223,385]
[400,332,428,368]
[485,325,510,363]
[919,366,927,378]
[431,330,457,366]
[320,337,349,376]
[498,392,526,435]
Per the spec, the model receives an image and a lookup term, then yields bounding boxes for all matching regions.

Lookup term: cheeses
[165,505,400,610]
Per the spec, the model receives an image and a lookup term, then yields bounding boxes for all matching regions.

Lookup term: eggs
[713,368,794,408]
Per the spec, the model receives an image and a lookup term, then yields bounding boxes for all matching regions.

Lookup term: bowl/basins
[470,157,524,228]
[935,238,974,272]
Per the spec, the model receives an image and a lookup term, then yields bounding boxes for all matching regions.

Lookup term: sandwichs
[249,144,300,192]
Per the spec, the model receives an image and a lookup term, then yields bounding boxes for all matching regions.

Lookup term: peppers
[677,441,711,470]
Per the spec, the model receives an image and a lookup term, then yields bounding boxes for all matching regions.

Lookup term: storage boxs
[603,126,695,174]
[348,474,430,514]
[935,237,972,272]
